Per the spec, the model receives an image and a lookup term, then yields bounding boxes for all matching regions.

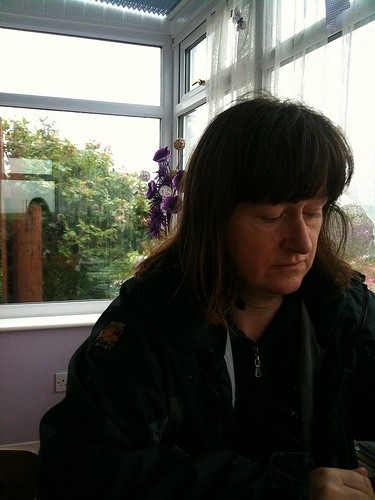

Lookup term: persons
[6,196,82,303]
[39,101,374,500]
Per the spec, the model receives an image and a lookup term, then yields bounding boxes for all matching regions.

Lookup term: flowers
[146,138,187,240]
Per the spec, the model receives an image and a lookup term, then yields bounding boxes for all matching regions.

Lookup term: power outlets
[55,373,68,393]
